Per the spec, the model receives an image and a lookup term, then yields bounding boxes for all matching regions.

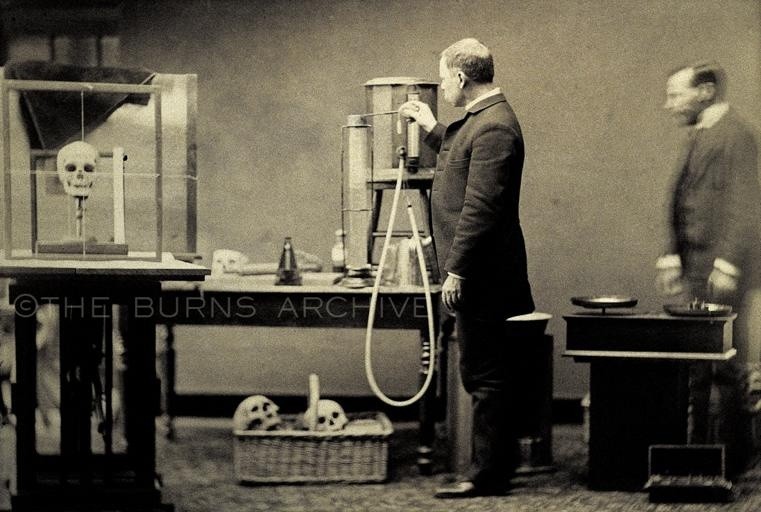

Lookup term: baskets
[234,373,394,483]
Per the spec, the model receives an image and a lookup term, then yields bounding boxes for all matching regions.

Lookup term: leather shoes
[434,479,476,497]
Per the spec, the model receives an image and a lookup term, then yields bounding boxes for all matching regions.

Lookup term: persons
[651,60,761,481]
[400,39,536,500]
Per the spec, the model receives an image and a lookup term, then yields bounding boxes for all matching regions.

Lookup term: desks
[160,273,447,475]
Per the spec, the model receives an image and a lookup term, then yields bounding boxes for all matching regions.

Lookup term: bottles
[329,229,347,273]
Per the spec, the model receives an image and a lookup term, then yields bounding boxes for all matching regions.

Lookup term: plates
[664,300,734,316]
